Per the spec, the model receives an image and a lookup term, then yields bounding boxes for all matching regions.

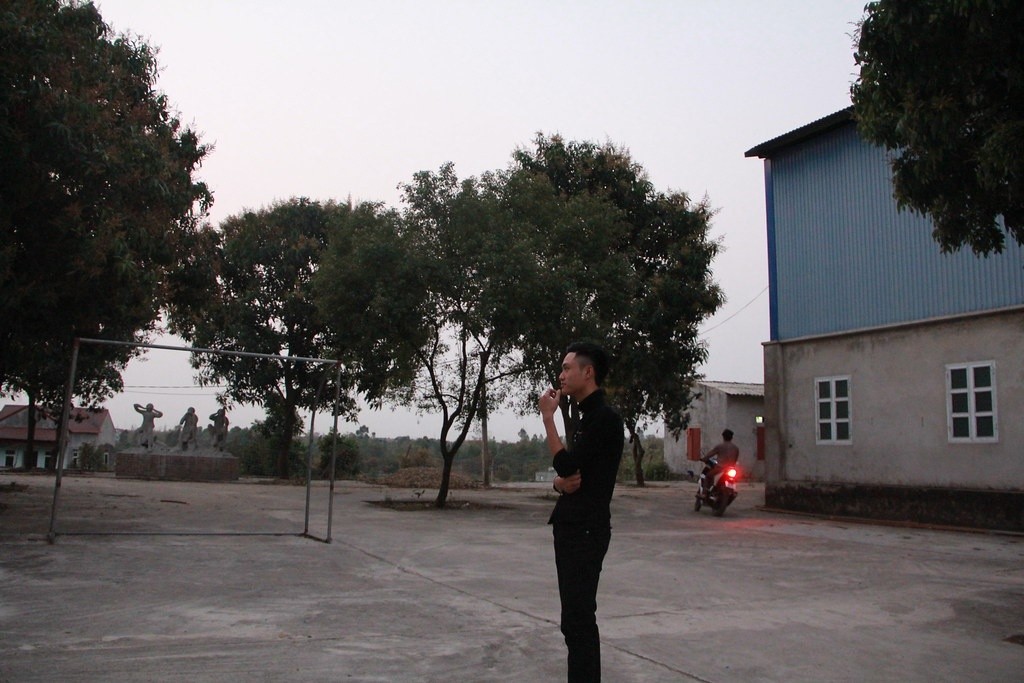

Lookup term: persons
[208,408,229,448]
[133,403,162,452]
[539,338,625,683]
[179,407,199,451]
[697,428,739,502]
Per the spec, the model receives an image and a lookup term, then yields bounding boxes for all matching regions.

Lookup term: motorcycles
[695,450,738,517]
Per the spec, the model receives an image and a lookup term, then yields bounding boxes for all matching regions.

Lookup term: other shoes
[701,488,708,497]
